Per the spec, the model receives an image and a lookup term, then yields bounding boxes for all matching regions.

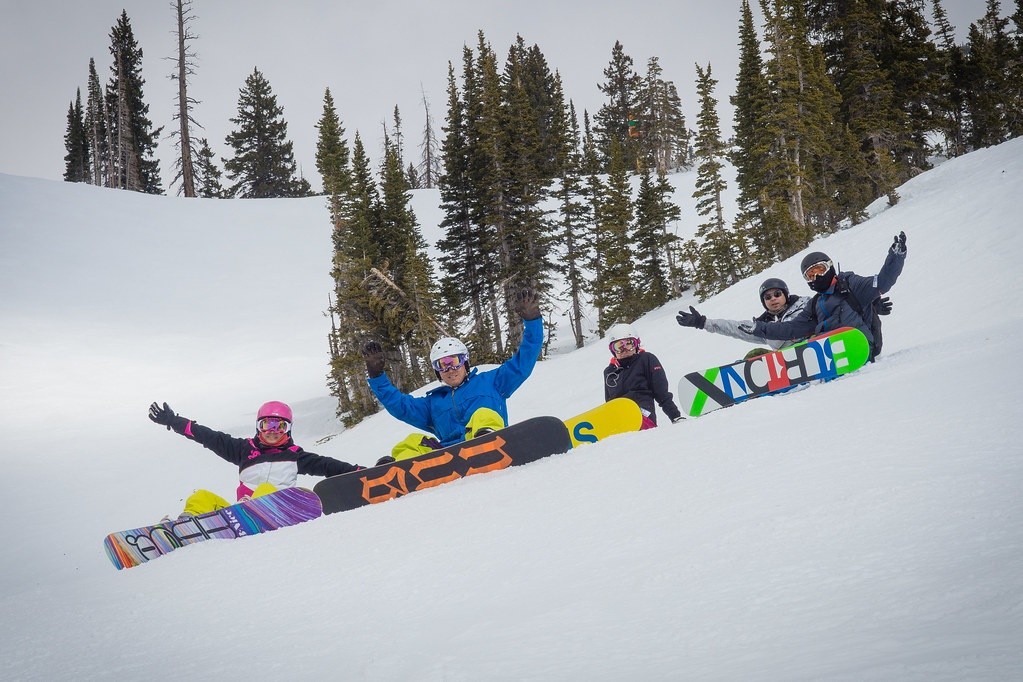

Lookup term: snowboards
[103,486,322,571]
[564,397,644,448]
[312,415,573,517]
[678,326,871,417]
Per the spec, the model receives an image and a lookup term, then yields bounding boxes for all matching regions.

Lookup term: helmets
[257,401,293,423]
[607,324,641,357]
[429,337,471,382]
[759,278,791,310]
[800,252,836,290]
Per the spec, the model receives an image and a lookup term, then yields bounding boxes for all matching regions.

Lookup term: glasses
[432,352,470,373]
[803,259,833,283]
[256,417,292,436]
[763,290,783,300]
[609,337,641,354]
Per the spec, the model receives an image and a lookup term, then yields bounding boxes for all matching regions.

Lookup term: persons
[148,401,366,523]
[604,324,685,430]
[738,231,907,359]
[361,290,543,465]
[676,278,892,350]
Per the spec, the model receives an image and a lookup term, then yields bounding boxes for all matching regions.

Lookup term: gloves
[514,290,541,321]
[876,297,893,315]
[738,316,760,334]
[362,342,385,377]
[891,231,907,251]
[148,402,190,436]
[676,305,706,330]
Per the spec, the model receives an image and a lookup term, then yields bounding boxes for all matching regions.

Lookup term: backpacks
[809,275,883,363]
